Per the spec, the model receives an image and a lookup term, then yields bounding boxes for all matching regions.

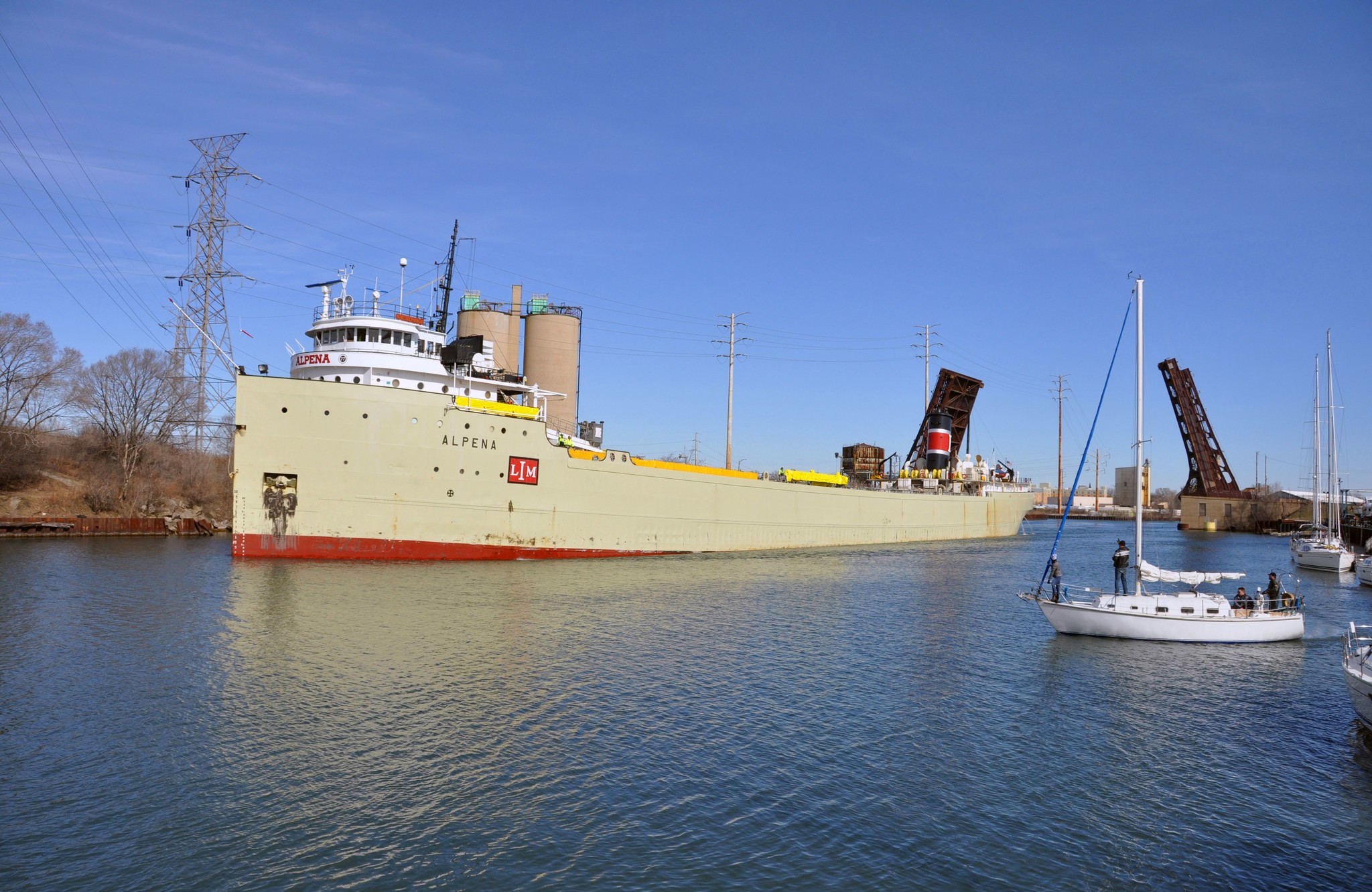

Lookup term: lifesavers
[962,474,967,479]
[1025,478,1027,482]
[1282,593,1294,608]
[920,473,925,478]
[879,473,885,478]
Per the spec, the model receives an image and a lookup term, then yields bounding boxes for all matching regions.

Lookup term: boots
[1051,594,1059,603]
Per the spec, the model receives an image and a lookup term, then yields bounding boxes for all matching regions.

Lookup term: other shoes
[1123,594,1128,596]
[1115,593,1118,596]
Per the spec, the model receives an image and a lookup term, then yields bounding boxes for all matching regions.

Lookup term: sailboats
[1014,277,1306,644]
[1290,327,1357,574]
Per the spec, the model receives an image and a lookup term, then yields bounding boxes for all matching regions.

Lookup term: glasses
[1237,591,1242,593]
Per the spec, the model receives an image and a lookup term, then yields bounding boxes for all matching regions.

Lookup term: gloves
[1047,562,1049,564]
[1047,580,1050,584]
[1117,538,1120,543]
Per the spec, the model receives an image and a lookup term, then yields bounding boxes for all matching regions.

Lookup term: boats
[1353,550,1372,586]
[1340,621,1372,732]
[229,219,1039,560]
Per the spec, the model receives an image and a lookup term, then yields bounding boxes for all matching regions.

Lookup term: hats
[1051,554,1057,560]
[1268,572,1276,577]
[1118,541,1125,546]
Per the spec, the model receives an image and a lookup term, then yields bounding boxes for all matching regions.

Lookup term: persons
[1234,587,1254,610]
[1112,538,1130,596]
[1261,572,1280,612]
[558,434,564,447]
[564,436,575,450]
[778,467,785,482]
[1047,554,1062,603]
